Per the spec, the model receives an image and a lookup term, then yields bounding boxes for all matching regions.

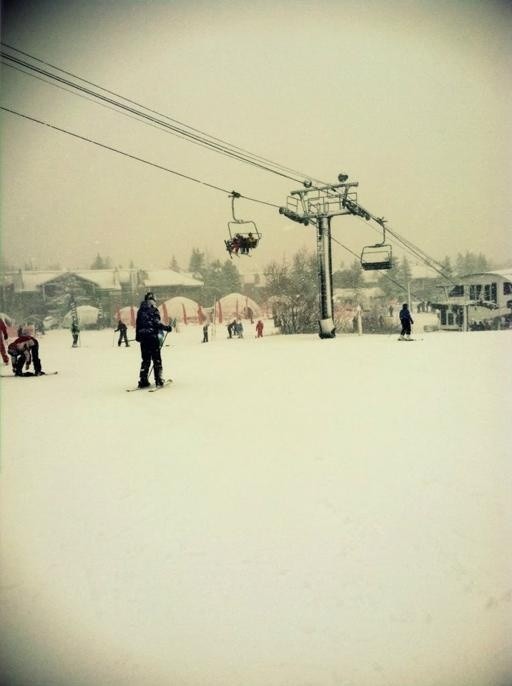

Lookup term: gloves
[162,325,172,332]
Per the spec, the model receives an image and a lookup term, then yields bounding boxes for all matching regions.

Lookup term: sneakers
[156,380,163,387]
[138,380,151,388]
[36,371,45,375]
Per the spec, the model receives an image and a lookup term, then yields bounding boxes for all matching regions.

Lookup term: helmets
[145,292,154,300]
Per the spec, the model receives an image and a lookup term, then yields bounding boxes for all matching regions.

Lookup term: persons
[228,233,244,256]
[232,320,239,335]
[417,301,424,313]
[8,335,46,377]
[237,322,244,338]
[71,318,81,348]
[135,291,173,389]
[426,301,430,312]
[113,320,131,347]
[389,304,393,317]
[0,318,9,364]
[17,325,23,338]
[201,320,212,343]
[255,320,264,338]
[228,322,235,338]
[172,318,177,327]
[241,231,257,254]
[399,303,414,340]
